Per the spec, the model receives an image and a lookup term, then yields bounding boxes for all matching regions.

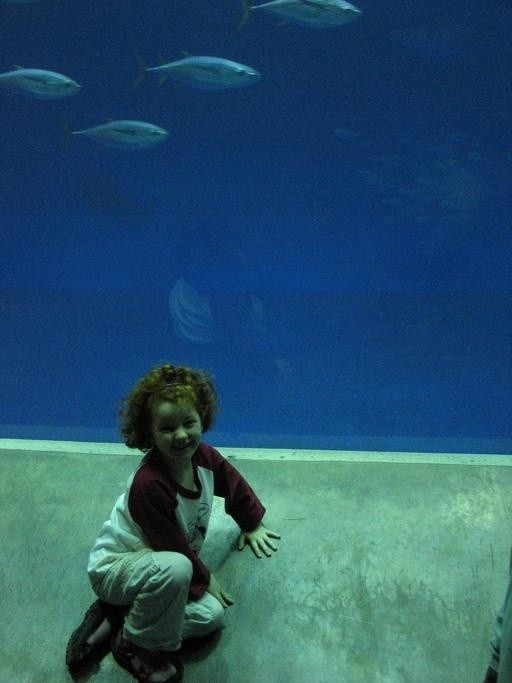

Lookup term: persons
[64,360,283,683]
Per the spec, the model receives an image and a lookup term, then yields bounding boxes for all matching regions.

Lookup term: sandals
[111,630,186,683]
[63,593,114,667]
